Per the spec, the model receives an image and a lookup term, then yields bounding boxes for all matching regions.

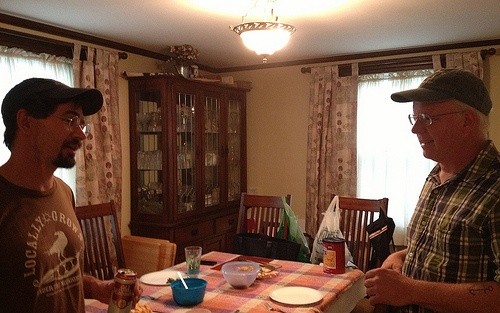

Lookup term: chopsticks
[177,272,188,290]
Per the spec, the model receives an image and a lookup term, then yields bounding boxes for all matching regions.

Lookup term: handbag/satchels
[234,232,301,261]
[278,196,310,263]
[309,195,355,270]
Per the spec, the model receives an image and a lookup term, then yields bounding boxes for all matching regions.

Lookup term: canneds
[107,268,137,313]
[321,237,345,274]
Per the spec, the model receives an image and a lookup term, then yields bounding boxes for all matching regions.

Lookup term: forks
[264,301,288,313]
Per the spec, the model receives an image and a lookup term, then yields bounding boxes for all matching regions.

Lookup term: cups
[185,246,202,276]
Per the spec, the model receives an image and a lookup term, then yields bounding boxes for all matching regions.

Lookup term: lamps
[229,0,296,64]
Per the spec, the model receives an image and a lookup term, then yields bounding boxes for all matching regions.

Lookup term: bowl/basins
[170,278,207,308]
[222,262,261,289]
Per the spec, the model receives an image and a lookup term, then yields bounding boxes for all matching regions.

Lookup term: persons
[357,68,500,313]
[0,77,143,313]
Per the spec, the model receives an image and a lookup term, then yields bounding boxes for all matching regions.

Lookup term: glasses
[407,109,468,124]
[41,112,90,134]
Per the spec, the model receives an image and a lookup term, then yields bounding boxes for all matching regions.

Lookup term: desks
[84,251,366,313]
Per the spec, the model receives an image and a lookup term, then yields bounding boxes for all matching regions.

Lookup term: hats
[1,78,104,125]
[391,68,492,116]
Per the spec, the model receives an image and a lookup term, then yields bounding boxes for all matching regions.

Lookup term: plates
[269,286,323,305]
[252,262,282,278]
[140,269,191,286]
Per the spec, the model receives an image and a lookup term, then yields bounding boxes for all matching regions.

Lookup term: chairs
[75,199,126,280]
[330,194,389,274]
[236,192,291,240]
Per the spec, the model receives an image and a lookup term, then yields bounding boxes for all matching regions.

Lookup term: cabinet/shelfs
[128,77,247,265]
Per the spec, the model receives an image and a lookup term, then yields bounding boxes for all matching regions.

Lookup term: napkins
[210,255,273,272]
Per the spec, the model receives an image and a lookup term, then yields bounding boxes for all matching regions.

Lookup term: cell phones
[200,260,217,266]
